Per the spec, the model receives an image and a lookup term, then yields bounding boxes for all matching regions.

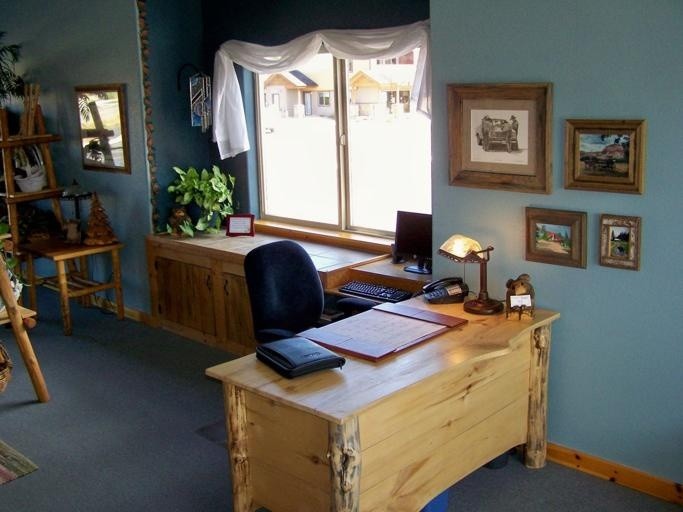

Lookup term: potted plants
[167,164,235,234]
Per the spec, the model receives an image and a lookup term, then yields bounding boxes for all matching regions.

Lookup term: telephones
[422,276,469,304]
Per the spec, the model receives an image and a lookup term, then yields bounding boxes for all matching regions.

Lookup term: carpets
[0,440,39,486]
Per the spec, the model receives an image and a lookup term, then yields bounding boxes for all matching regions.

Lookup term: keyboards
[339,281,412,303]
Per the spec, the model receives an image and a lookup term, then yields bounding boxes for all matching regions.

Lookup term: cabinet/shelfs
[0,233,51,403]
[0,105,82,326]
[146,223,392,357]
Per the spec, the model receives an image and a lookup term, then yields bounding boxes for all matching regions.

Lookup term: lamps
[437,234,504,315]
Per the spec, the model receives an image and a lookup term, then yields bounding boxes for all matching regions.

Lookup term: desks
[324,256,432,305]
[206,294,560,512]
[17,237,126,336]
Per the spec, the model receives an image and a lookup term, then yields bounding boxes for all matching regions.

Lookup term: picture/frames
[446,83,552,195]
[564,119,646,195]
[525,207,586,269]
[226,214,255,236]
[600,214,641,271]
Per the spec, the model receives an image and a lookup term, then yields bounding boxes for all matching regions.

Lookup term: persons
[614,240,624,257]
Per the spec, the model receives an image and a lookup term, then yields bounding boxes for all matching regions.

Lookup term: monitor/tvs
[395,211,432,274]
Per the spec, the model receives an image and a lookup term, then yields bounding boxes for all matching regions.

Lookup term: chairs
[244,240,325,343]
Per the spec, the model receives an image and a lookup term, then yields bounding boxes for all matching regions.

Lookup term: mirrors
[75,84,132,174]
